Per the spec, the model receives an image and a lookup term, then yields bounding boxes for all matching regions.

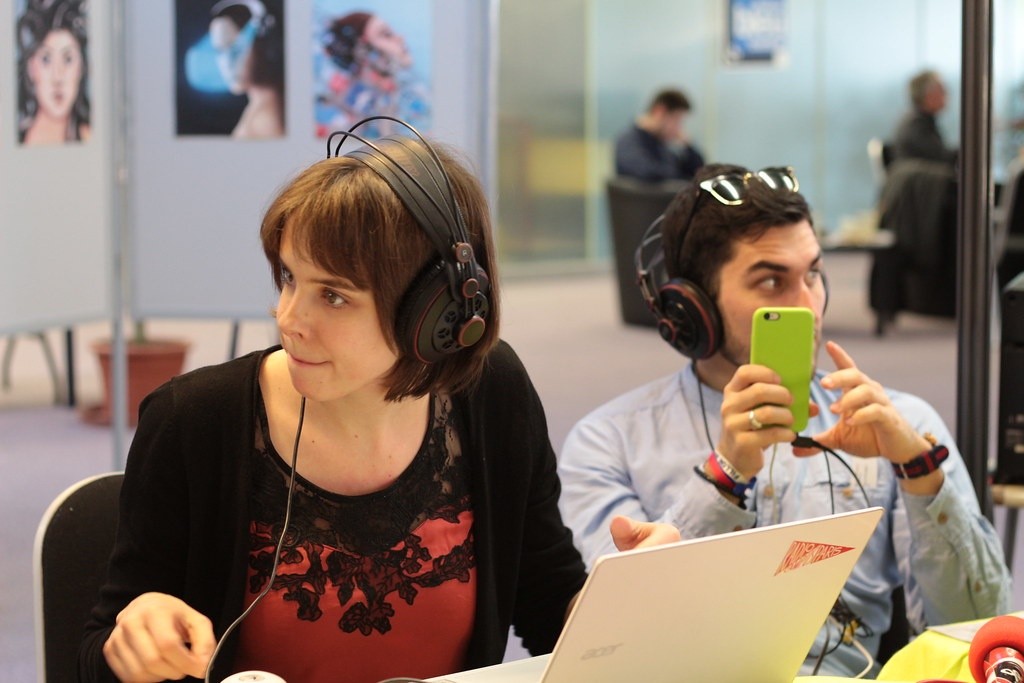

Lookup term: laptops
[422,506,885,683]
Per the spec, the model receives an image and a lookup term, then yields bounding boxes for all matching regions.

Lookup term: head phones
[637,214,724,361]
[342,133,491,364]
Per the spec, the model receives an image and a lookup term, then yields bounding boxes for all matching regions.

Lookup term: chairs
[604,173,697,329]
[29,468,138,683]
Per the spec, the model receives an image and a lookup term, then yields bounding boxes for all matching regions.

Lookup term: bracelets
[892,434,948,481]
[704,452,757,501]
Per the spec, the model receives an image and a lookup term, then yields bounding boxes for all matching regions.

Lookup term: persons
[889,69,961,164]
[610,88,705,182]
[554,163,1013,682]
[73,136,687,683]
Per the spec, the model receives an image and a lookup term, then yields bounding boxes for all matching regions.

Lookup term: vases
[94,338,189,430]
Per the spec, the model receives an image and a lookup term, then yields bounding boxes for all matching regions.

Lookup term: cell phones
[750,306,816,432]
